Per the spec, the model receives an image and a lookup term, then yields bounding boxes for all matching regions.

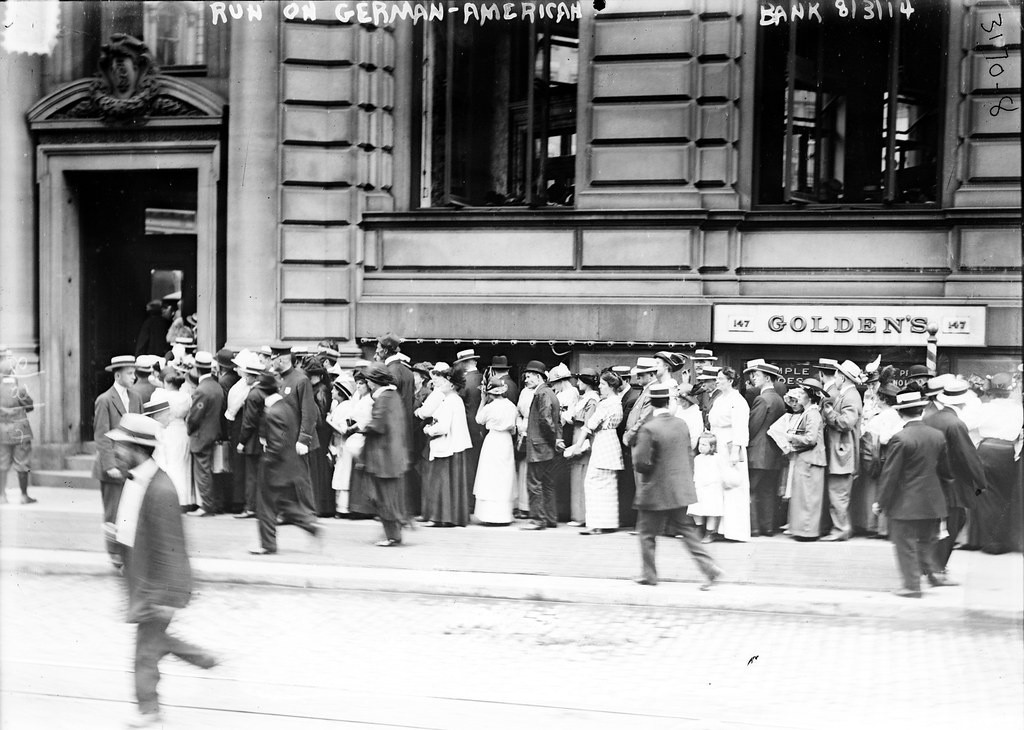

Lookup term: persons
[93,343,1024,599]
[0,345,37,504]
[104,414,233,728]
[128,303,198,356]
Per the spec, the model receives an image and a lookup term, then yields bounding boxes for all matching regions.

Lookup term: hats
[892,391,930,410]
[186,312,198,326]
[103,412,164,447]
[105,344,328,376]
[411,348,1024,404]
[334,375,358,400]
[141,397,170,415]
[170,337,198,348]
[377,330,401,352]
[361,362,394,384]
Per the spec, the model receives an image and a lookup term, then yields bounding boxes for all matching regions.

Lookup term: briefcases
[212,442,231,473]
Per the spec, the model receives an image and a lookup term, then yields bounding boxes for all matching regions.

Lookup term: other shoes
[187,509,215,516]
[519,523,546,530]
[376,540,402,547]
[820,535,846,541]
[634,578,657,585]
[894,588,922,599]
[779,523,791,536]
[233,510,258,520]
[567,521,586,527]
[580,528,602,534]
[249,547,276,554]
[128,712,162,727]
[930,575,959,587]
[700,571,724,590]
[702,531,717,543]
[415,515,442,527]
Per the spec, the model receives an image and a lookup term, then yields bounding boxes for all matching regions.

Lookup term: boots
[0,469,37,506]
